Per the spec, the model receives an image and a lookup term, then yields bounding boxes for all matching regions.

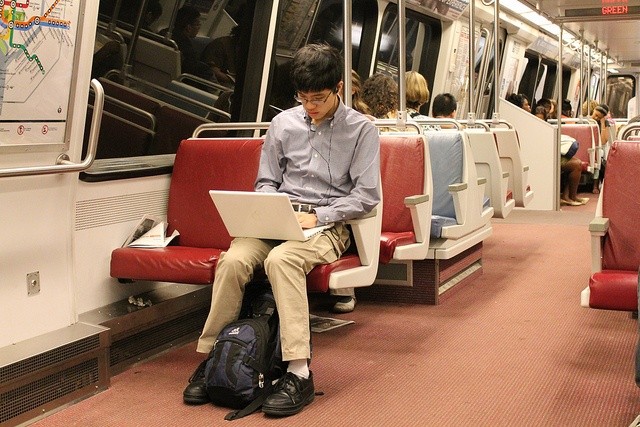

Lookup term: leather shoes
[263,372,314,416]
[182,359,205,405]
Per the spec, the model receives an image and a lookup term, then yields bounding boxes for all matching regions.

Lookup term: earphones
[333,86,339,93]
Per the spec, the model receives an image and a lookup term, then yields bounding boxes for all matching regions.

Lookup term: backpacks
[205,296,278,421]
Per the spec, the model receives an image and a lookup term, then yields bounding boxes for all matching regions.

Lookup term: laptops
[209,189,324,241]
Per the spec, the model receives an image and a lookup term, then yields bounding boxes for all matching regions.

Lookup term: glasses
[293,90,334,104]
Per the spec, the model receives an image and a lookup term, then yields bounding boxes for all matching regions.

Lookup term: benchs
[467,131,495,223]
[588,119,601,170]
[374,119,434,262]
[110,122,384,289]
[548,118,595,174]
[416,118,473,240]
[461,121,515,218]
[580,125,637,309]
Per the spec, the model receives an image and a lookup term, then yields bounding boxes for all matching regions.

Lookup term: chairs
[97,23,284,150]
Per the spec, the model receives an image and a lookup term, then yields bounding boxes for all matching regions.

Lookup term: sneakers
[334,297,356,313]
[582,197,589,204]
[560,199,581,206]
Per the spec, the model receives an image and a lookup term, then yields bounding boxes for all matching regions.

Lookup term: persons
[330,67,371,315]
[507,91,615,207]
[401,72,430,114]
[433,91,459,123]
[362,71,400,119]
[185,39,379,413]
